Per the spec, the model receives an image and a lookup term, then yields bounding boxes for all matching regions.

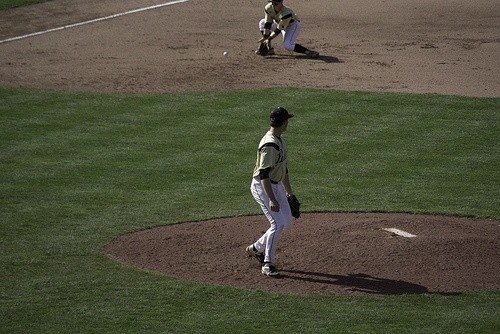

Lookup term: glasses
[272,3,279,5]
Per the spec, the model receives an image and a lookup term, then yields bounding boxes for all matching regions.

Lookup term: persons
[256,0,320,58]
[246,107,301,277]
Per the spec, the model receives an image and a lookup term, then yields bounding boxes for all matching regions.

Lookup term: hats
[269,0,283,3]
[270,106,294,121]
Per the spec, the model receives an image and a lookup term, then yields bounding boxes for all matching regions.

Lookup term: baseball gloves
[286,194,303,220]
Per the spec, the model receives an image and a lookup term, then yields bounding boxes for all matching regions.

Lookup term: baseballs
[224,52,228,56]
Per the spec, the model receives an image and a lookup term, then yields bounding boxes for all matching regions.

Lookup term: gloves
[257,34,269,44]
[263,35,273,51]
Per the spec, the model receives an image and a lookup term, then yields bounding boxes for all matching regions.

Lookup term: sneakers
[245,244,265,267]
[261,261,281,278]
[311,52,319,60]
